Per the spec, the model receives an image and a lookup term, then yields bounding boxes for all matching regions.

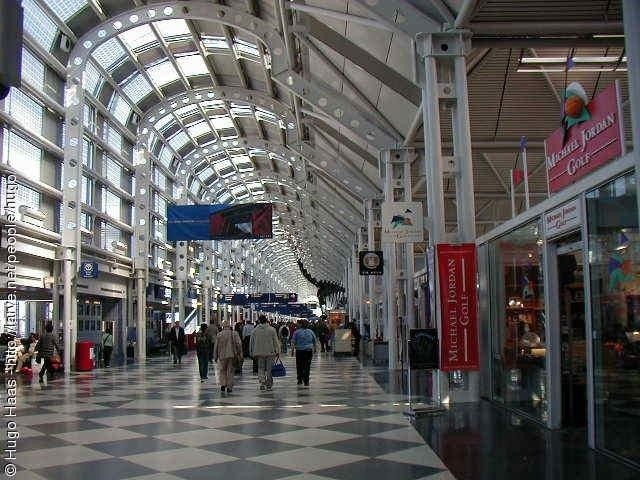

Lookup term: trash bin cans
[76,340,96,371]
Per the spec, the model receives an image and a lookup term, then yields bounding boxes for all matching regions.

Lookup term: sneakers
[220,380,310,393]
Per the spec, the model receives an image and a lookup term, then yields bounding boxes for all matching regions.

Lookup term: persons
[102,328,113,367]
[291,320,318,386]
[248,316,286,390]
[191,315,331,374]
[196,323,213,383]
[35,324,60,385]
[171,321,186,364]
[213,320,244,392]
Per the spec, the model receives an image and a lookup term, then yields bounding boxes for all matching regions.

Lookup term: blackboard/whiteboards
[409,328,439,370]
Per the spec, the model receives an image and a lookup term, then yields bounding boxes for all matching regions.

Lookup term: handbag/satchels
[35,353,42,364]
[272,356,286,378]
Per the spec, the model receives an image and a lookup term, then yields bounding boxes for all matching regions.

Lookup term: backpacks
[282,326,289,337]
